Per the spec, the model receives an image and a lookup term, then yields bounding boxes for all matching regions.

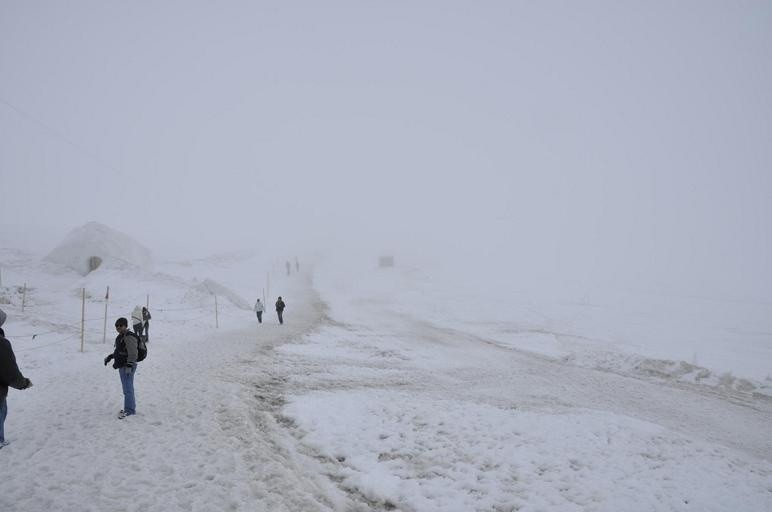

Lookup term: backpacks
[126,332,147,362]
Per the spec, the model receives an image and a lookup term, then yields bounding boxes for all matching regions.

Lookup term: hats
[0,309,7,327]
[114,318,128,327]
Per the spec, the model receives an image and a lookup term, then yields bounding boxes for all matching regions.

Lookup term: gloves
[105,355,113,365]
[24,378,32,389]
[125,363,133,376]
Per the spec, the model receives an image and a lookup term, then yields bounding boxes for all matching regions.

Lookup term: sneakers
[119,410,130,419]
[0,440,9,448]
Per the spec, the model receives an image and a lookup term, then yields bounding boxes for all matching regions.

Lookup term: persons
[253,297,266,323]
[276,296,286,324]
[143,306,152,341]
[104,317,148,419]
[131,304,144,336]
[0,309,33,448]
